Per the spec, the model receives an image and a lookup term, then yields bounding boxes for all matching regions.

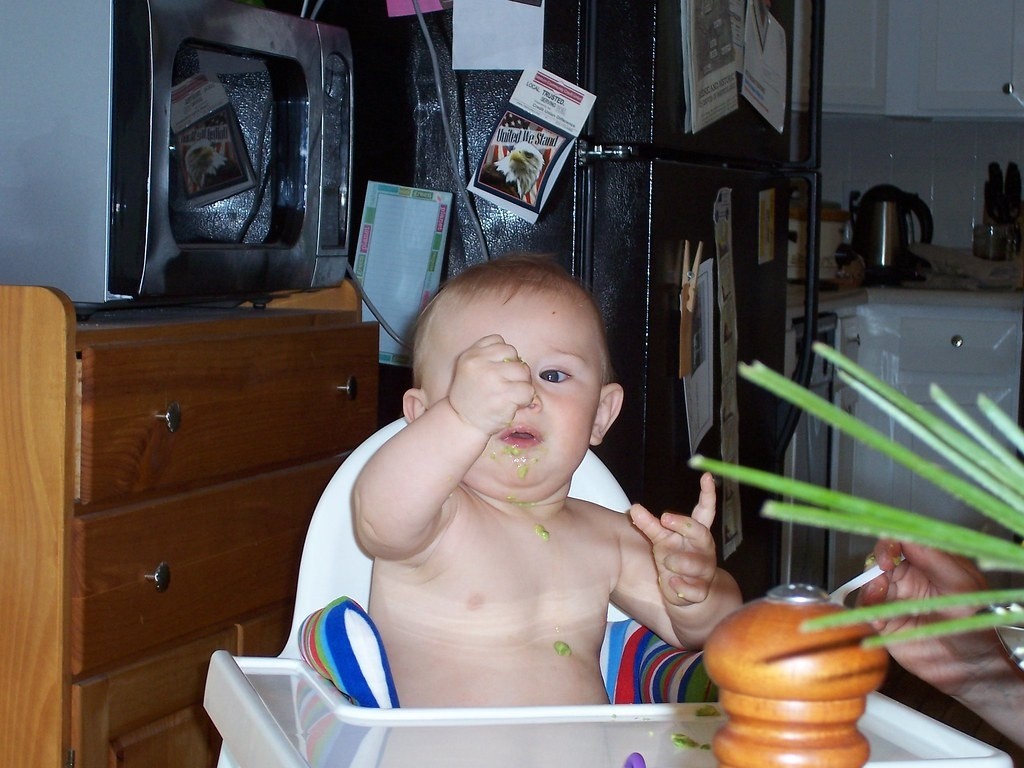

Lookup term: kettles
[851,184,935,287]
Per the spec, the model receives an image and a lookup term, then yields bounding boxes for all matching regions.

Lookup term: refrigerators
[410,0,827,605]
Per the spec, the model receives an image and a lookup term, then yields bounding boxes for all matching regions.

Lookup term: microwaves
[0,1,409,309]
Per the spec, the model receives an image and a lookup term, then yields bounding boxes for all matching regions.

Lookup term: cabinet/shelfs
[855,310,1022,553]
[0,281,378,768]
[822,0,1024,121]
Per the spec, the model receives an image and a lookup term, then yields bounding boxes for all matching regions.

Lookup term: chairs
[202,414,1014,768]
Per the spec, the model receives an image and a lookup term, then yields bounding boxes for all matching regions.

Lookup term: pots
[787,175,852,291]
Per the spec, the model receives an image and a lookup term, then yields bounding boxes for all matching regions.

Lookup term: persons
[355,251,743,710]
[856,539,1024,750]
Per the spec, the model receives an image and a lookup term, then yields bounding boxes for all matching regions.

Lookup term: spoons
[827,548,906,608]
